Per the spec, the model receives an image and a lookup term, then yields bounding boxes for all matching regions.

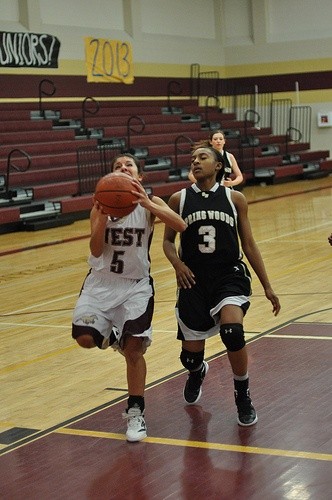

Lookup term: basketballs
[95,172,139,218]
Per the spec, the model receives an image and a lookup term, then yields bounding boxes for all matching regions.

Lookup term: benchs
[0,96,332,230]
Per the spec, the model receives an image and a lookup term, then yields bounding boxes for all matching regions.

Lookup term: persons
[188,130,242,190]
[72,153,187,441]
[163,146,280,426]
[328,233,332,245]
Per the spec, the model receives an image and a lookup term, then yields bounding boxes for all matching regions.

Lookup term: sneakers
[123,408,147,442]
[233,378,258,426]
[183,360,209,404]
[111,323,126,357]
